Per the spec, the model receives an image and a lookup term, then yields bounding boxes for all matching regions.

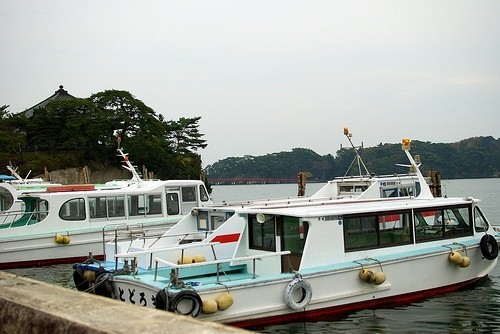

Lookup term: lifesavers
[480,234,499,261]
[156,284,202,319]
[283,277,314,312]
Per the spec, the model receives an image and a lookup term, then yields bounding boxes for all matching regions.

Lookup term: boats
[212,173,443,201]
[0,179,211,260]
[72,196,499,328]
